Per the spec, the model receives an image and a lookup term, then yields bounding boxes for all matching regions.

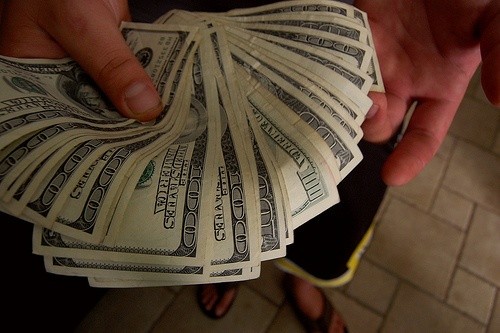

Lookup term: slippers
[281,271,348,332]
[193,279,239,320]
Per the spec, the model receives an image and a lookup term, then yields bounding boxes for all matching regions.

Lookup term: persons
[1,0,500,333]
